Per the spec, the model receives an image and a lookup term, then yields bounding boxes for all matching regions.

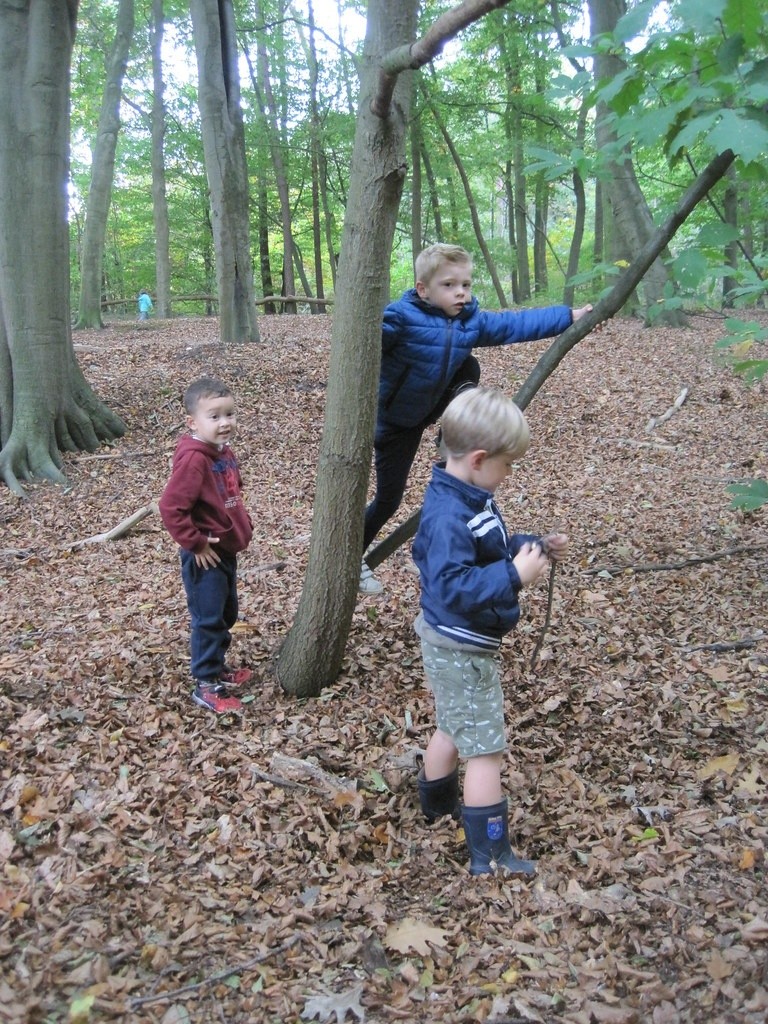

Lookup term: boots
[461,797,539,877]
[418,759,463,825]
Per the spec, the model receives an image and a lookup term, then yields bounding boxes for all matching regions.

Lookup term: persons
[159,378,254,713]
[413,387,571,875]
[136,288,154,320]
[356,244,606,597]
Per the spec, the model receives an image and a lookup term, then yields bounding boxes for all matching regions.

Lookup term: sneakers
[214,666,251,686]
[192,681,242,714]
[356,558,382,594]
